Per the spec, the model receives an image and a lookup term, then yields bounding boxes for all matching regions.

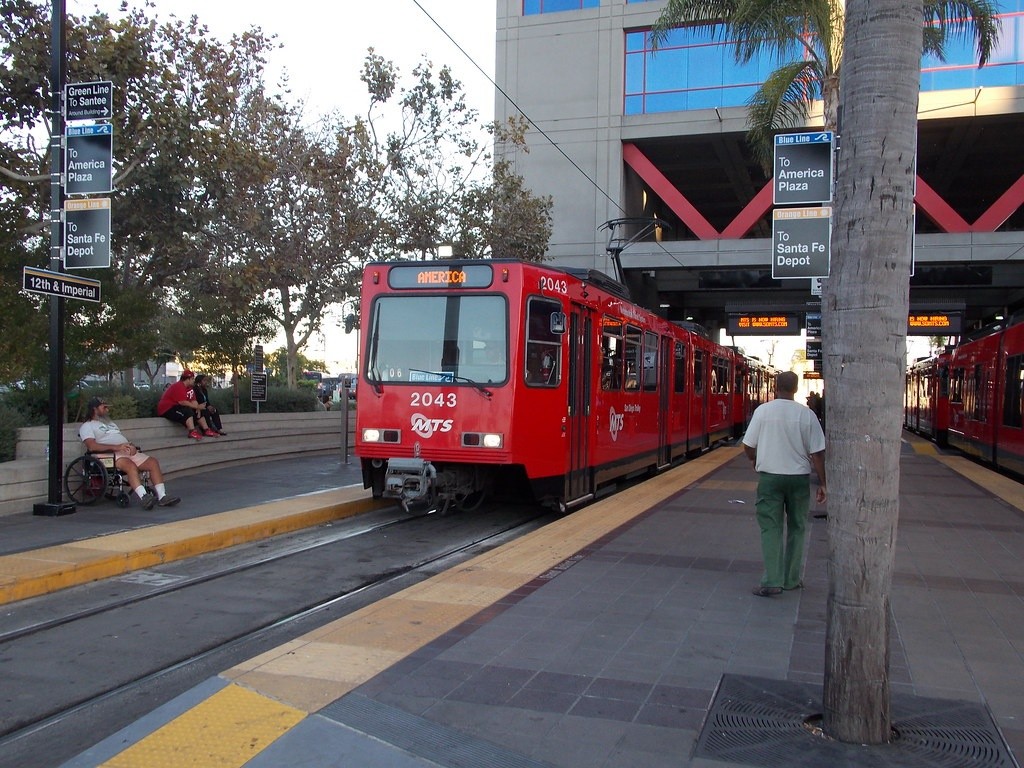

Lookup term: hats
[88,396,114,408]
[183,369,194,377]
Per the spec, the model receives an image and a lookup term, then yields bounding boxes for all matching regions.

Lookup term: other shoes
[215,430,227,436]
[204,427,220,437]
[783,580,805,590]
[158,495,182,506]
[752,584,785,597]
[140,494,159,511]
[187,428,204,440]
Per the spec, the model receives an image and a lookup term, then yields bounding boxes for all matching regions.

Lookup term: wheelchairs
[64,434,159,507]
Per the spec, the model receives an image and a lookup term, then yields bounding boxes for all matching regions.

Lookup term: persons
[79,397,181,511]
[191,374,228,436]
[157,369,220,440]
[807,391,822,419]
[742,371,827,597]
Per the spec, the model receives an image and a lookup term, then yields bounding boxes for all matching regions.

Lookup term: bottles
[45,443,49,461]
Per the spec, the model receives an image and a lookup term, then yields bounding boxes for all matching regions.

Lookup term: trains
[343,255,782,511]
[904,314,1024,477]
